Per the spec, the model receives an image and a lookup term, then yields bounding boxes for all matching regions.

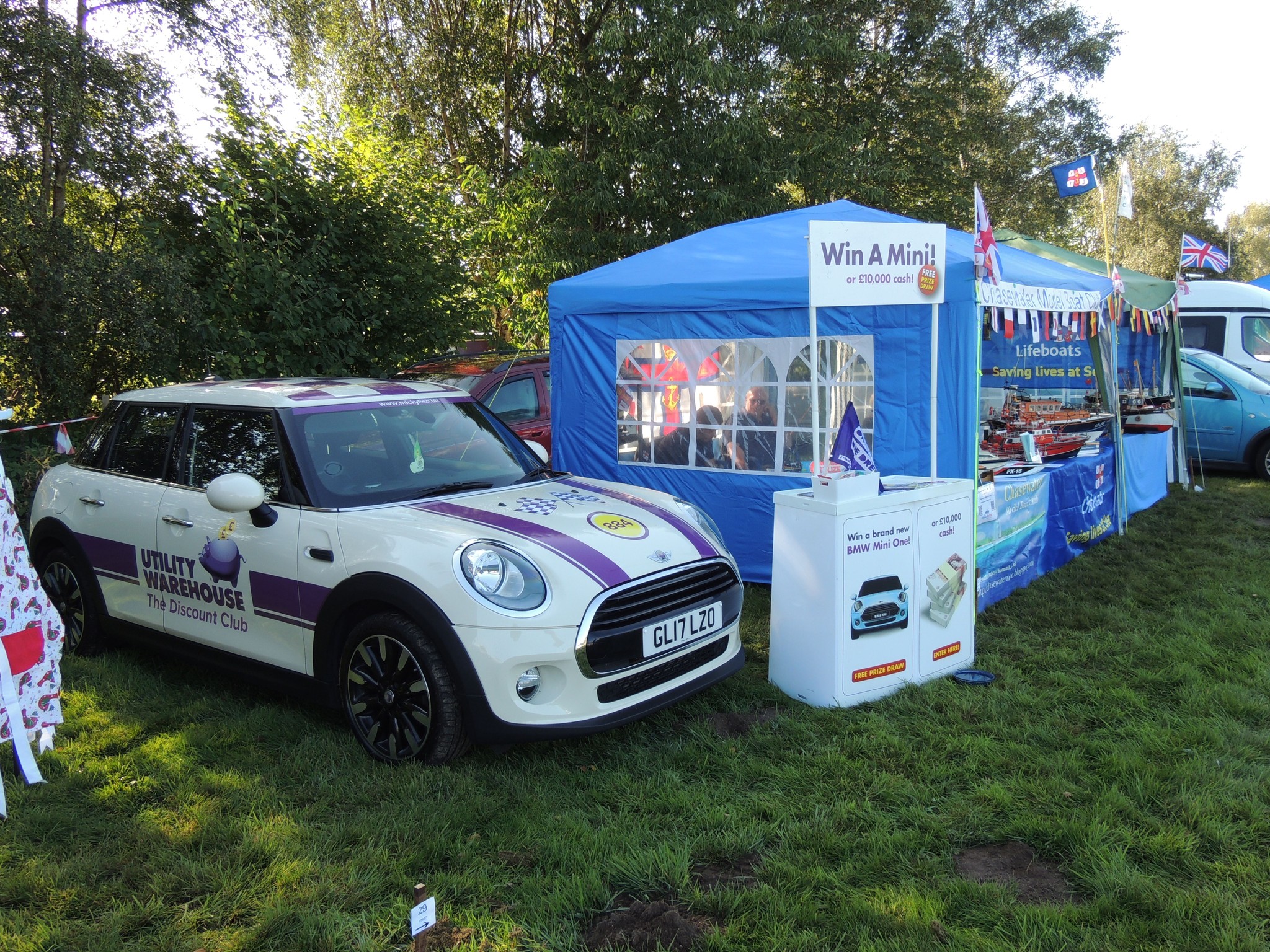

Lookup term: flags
[975,187,1004,333]
[1049,155,1100,197]
[828,401,884,496]
[1181,235,1229,274]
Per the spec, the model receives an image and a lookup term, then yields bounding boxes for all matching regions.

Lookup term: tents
[546,198,1191,618]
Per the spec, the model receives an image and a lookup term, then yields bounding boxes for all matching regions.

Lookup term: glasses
[749,399,766,405]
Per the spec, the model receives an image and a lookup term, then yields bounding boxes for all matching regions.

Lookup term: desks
[977,432,1167,612]
[768,475,974,709]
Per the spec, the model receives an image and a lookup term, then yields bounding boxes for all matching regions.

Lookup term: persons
[656,405,723,469]
[722,386,792,471]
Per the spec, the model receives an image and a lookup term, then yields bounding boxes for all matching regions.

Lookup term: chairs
[314,414,385,488]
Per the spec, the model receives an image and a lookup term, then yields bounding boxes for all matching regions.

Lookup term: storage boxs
[811,470,880,504]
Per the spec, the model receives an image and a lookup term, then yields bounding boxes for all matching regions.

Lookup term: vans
[1171,280,1268,381]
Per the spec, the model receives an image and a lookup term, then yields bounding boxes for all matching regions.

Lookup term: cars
[1178,346,1269,478]
[390,355,636,464]
[24,372,745,762]
